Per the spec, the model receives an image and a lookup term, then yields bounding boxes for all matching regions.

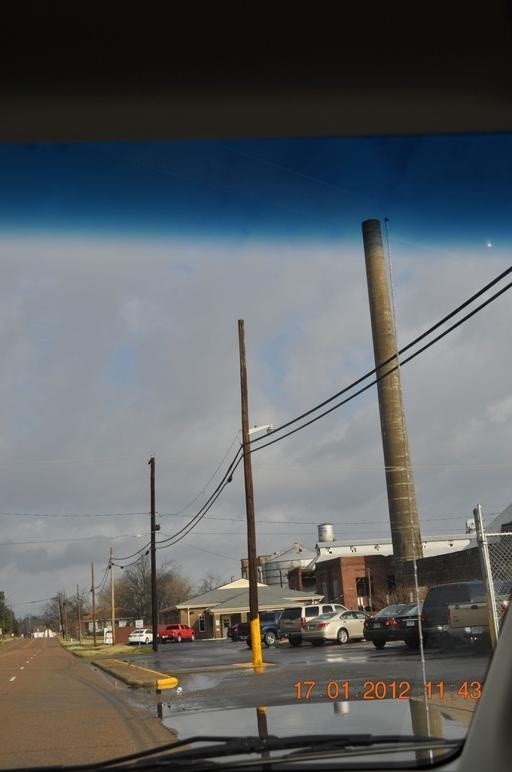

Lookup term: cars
[127,629,153,644]
[226,580,512,656]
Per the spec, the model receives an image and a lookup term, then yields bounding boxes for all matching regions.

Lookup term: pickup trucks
[159,625,195,643]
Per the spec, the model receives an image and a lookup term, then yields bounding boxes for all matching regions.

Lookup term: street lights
[236,422,276,675]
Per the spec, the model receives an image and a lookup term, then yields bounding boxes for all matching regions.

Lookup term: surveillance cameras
[266,426,276,433]
